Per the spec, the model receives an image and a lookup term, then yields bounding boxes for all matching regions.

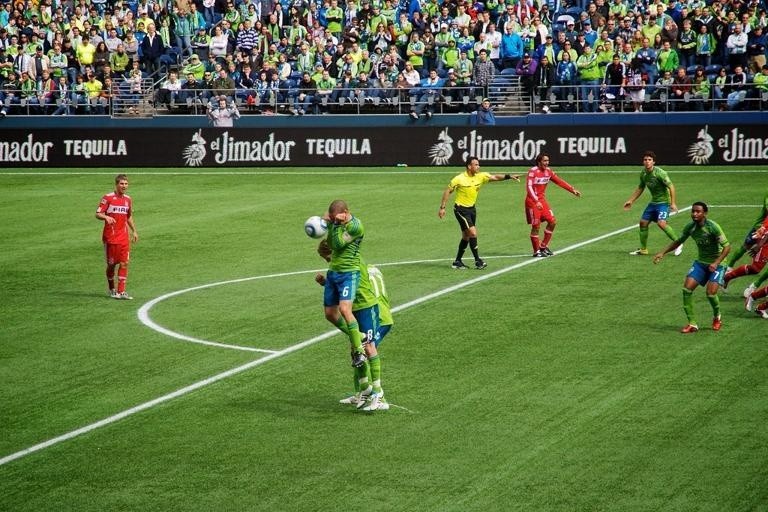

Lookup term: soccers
[306,216,329,240]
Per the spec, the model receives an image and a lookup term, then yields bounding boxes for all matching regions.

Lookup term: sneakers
[532,250,547,257]
[453,261,469,269]
[541,246,553,255]
[543,106,548,112]
[629,249,648,255]
[675,244,682,255]
[262,97,431,120]
[340,333,389,411]
[474,262,487,270]
[110,289,120,299]
[681,268,768,332]
[119,292,133,300]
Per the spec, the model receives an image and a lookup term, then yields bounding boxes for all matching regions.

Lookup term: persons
[313,199,393,412]
[95,174,137,300]
[654,201,729,334]
[525,152,581,257]
[1,0,768,127]
[439,156,521,271]
[623,151,683,257]
[722,194,768,320]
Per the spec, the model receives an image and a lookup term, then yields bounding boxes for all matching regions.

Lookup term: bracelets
[439,206,445,210]
[505,174,510,180]
